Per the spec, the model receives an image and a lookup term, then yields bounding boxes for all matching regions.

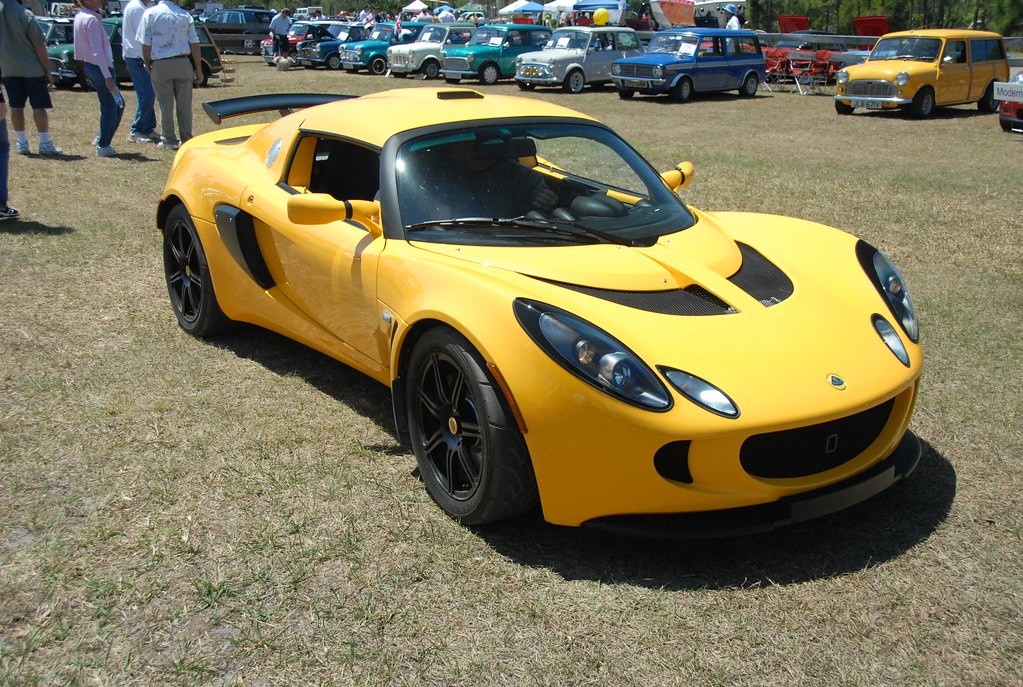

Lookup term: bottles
[111,87,124,108]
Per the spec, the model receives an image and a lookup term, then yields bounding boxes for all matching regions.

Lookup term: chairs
[763,47,842,98]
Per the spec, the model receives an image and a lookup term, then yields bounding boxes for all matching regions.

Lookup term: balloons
[593,8,610,25]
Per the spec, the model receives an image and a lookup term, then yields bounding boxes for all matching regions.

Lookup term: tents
[433,5,458,15]
[544,0,631,12]
[457,5,485,12]
[402,0,428,12]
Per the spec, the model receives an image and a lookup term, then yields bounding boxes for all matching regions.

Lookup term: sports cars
[153,85,924,546]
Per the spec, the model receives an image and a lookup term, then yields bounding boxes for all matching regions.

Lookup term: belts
[154,55,189,61]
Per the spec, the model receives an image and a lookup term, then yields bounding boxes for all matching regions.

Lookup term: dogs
[273,52,297,72]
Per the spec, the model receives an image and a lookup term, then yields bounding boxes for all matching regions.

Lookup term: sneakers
[92,138,117,157]
[39,140,63,154]
[179,141,184,146]
[127,132,165,143]
[0,206,20,218]
[17,140,31,154]
[156,141,177,149]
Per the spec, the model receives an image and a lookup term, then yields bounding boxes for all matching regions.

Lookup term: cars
[26,8,768,104]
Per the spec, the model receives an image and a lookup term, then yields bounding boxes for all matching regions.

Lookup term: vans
[834,27,1012,120]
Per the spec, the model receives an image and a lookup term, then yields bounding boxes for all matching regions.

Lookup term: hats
[722,4,736,14]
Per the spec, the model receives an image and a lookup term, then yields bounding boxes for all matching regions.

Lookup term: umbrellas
[499,0,544,14]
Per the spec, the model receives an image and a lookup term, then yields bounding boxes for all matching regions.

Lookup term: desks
[788,51,843,95]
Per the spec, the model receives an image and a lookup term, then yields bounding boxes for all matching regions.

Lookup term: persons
[72,0,125,157]
[121,0,161,143]
[545,14,552,29]
[269,8,291,59]
[0,66,20,218]
[308,3,486,27]
[135,0,204,149]
[465,157,561,218]
[0,0,63,156]
[722,4,742,52]
